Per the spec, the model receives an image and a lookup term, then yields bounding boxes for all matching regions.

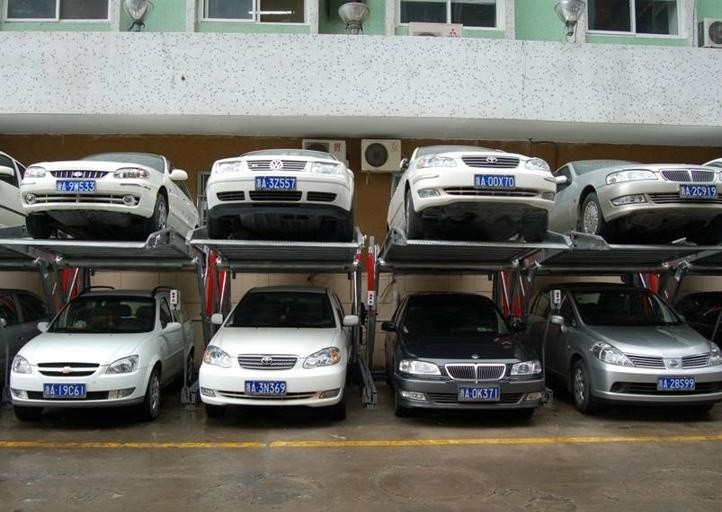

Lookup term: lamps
[337,2,370,35]
[123,0,155,32]
[553,0,586,42]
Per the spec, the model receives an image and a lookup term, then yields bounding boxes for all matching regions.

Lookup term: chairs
[596,293,624,318]
[114,305,136,325]
[134,305,155,327]
[84,306,111,326]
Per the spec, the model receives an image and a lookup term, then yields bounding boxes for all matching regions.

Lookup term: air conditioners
[301,139,347,168]
[360,138,402,174]
[409,21,465,42]
[698,18,721,47]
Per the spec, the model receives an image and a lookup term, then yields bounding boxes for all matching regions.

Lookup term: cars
[0,286,51,409]
[381,291,548,422]
[547,157,720,243]
[10,285,195,424]
[17,150,199,245]
[386,144,568,243]
[0,150,68,240]
[666,250,722,351]
[524,281,722,415]
[205,147,357,244]
[196,285,359,421]
